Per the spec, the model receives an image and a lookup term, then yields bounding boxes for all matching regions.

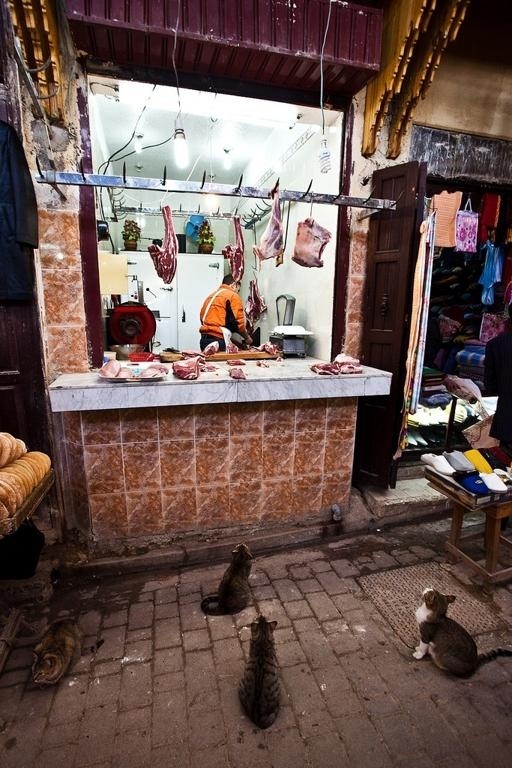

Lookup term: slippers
[421,445,512,494]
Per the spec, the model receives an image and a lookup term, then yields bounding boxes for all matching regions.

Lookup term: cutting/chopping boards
[204,352,283,360]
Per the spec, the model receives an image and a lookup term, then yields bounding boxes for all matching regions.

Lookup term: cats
[412,587,512,680]
[30,618,104,690]
[201,542,255,616]
[238,613,281,729]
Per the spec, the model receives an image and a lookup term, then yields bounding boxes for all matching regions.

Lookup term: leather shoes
[408,430,441,447]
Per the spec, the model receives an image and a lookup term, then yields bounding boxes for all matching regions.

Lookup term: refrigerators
[123,251,224,352]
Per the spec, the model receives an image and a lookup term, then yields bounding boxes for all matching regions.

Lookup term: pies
[0,432,51,520]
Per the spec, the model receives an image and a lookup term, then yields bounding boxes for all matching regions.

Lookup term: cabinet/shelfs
[0,469,58,672]
[446,498,512,603]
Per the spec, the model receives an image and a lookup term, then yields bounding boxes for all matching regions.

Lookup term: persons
[200,274,252,353]
[483,301,512,460]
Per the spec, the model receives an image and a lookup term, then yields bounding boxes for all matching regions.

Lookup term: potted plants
[196,221,216,253]
[121,219,141,250]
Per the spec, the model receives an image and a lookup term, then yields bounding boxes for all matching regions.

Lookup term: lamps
[174,120,190,169]
[319,134,330,171]
